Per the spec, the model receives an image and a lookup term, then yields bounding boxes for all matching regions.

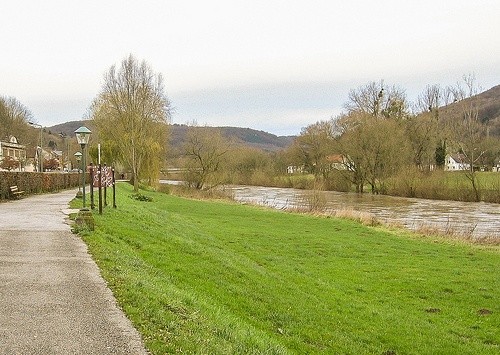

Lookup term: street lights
[74,151,84,199]
[75,125,92,214]
[27,120,44,174]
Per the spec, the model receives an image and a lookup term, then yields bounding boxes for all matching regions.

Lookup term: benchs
[8,185,25,198]
